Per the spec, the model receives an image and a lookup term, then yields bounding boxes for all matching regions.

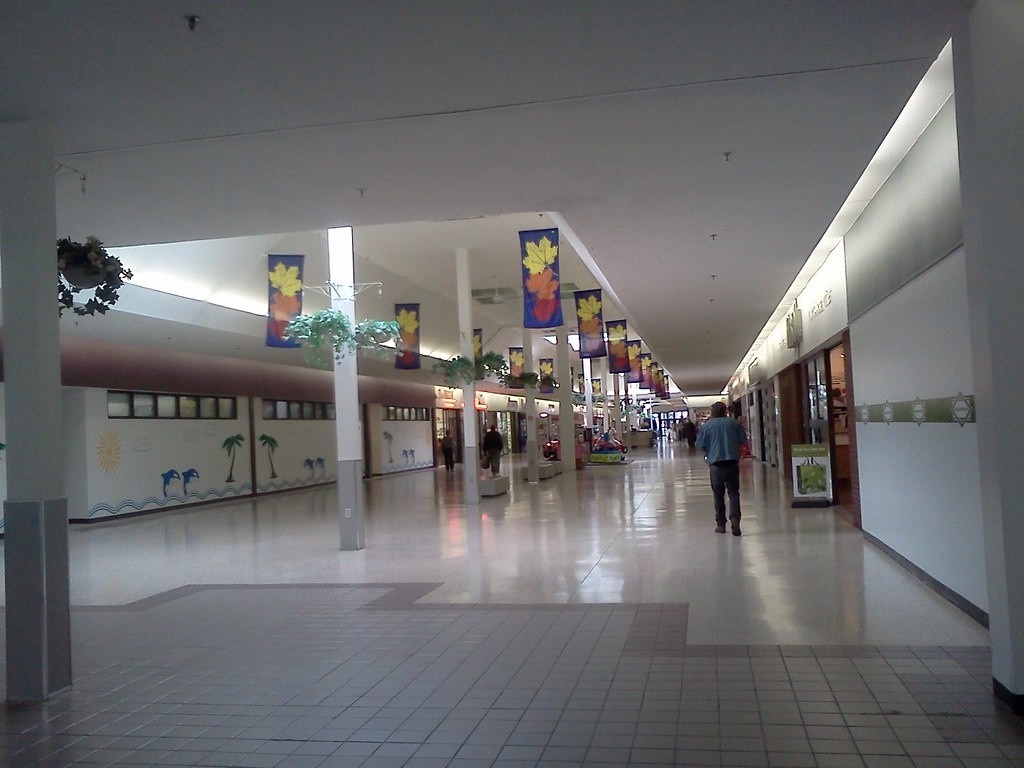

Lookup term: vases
[62,267,104,289]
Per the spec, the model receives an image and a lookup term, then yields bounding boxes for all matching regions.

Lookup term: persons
[695,402,746,535]
[483,423,503,474]
[439,430,456,472]
[677,420,682,440]
[521,426,527,453]
[437,438,443,464]
[684,418,695,448]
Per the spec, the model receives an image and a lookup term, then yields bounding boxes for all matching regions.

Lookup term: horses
[594,426,622,451]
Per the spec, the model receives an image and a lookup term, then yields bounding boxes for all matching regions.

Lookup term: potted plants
[434,350,553,391]
[285,308,355,365]
[355,318,405,356]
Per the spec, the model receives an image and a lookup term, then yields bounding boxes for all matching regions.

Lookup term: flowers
[56,235,133,319]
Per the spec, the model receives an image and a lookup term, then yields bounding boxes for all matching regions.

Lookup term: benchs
[522,463,555,479]
[479,474,511,496]
[547,461,562,473]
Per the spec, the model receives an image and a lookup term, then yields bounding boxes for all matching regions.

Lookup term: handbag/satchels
[481,456,490,470]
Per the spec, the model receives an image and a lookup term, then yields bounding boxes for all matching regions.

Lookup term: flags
[570,367,601,396]
[395,304,420,369]
[639,354,671,400]
[473,329,484,380]
[573,289,606,358]
[539,359,553,393]
[605,321,630,373]
[508,348,525,388]
[265,255,303,348]
[626,340,643,382]
[519,229,563,328]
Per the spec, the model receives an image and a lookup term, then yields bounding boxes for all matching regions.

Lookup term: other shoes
[731,518,741,537]
[713,520,726,534]
